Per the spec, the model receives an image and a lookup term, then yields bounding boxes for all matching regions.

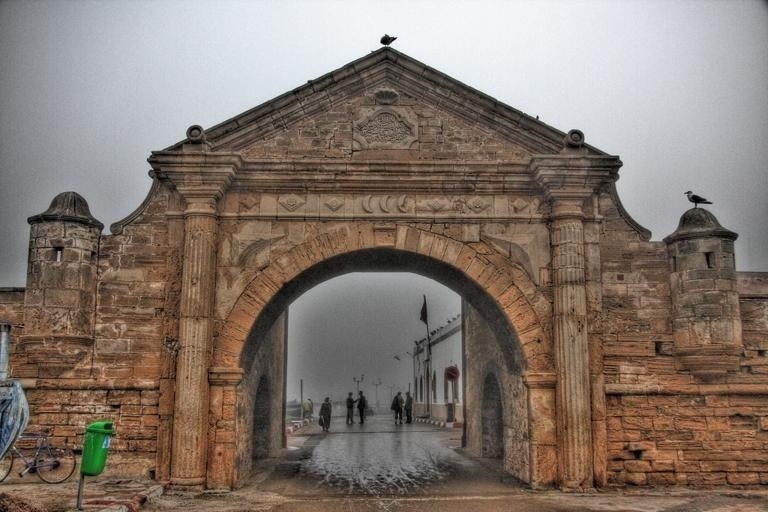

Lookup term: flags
[419,296,426,323]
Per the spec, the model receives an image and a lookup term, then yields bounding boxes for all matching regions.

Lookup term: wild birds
[682,189,712,207]
[380,33,398,47]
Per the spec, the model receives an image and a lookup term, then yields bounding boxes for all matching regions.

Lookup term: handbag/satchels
[318,415,324,426]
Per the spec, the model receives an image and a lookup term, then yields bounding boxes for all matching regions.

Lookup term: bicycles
[0,425,77,484]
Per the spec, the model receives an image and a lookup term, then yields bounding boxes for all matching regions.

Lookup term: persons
[394,391,404,424]
[345,392,356,424]
[357,391,365,424]
[318,397,332,432]
[303,399,312,417]
[403,392,412,423]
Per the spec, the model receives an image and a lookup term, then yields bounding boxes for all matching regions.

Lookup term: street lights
[372,377,382,407]
[352,373,365,416]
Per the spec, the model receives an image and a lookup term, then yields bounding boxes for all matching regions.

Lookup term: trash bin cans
[81,420,114,476]
[445,403,457,422]
